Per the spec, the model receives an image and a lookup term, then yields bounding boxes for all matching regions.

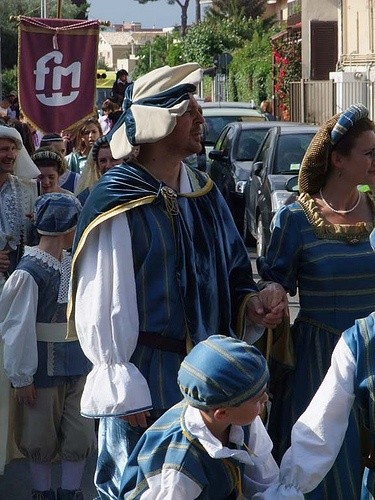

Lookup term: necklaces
[320,187,360,213]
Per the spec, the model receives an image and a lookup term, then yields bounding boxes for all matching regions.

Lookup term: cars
[209,120,309,223]
[244,126,321,255]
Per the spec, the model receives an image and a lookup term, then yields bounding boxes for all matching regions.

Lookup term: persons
[259,104,375,500]
[0,69,133,500]
[116,335,280,500]
[253,312,375,500]
[65,61,284,500]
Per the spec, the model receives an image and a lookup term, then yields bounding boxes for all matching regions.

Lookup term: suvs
[198,95,258,110]
[198,110,278,175]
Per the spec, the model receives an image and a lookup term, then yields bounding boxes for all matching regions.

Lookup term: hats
[31,192,81,235]
[177,334,269,410]
[0,125,41,181]
[106,62,204,159]
[8,91,17,97]
